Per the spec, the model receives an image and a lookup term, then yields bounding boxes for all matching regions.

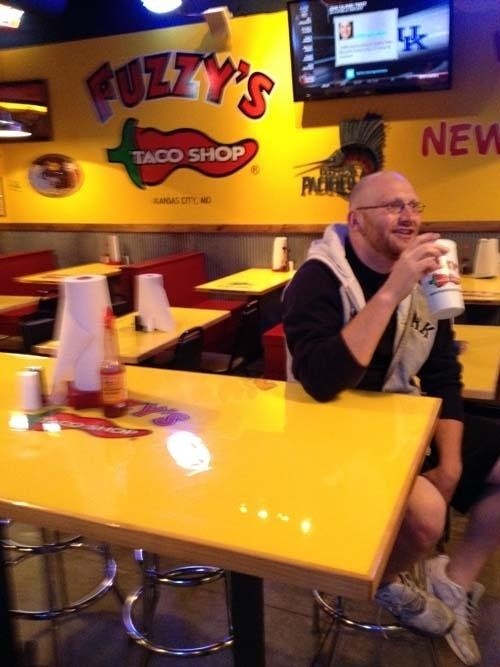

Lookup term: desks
[0,261,500,666]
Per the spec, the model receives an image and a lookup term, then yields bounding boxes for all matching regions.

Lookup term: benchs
[1,247,59,336]
[119,251,248,366]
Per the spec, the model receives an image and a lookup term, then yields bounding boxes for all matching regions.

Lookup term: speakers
[204,6,231,39]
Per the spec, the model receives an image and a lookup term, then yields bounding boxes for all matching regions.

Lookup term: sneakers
[374,570,458,641]
[422,551,485,666]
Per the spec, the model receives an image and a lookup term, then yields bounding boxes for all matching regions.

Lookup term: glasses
[356,200,427,214]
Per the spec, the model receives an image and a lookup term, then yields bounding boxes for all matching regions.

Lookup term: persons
[282,170,500,666]
[339,19,354,40]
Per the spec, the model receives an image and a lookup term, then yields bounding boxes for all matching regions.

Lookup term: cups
[416,239,465,321]
[17,364,48,411]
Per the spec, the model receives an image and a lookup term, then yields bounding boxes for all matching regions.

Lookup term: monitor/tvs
[287,0,453,102]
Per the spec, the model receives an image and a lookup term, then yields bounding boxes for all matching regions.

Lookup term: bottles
[100,306,127,419]
